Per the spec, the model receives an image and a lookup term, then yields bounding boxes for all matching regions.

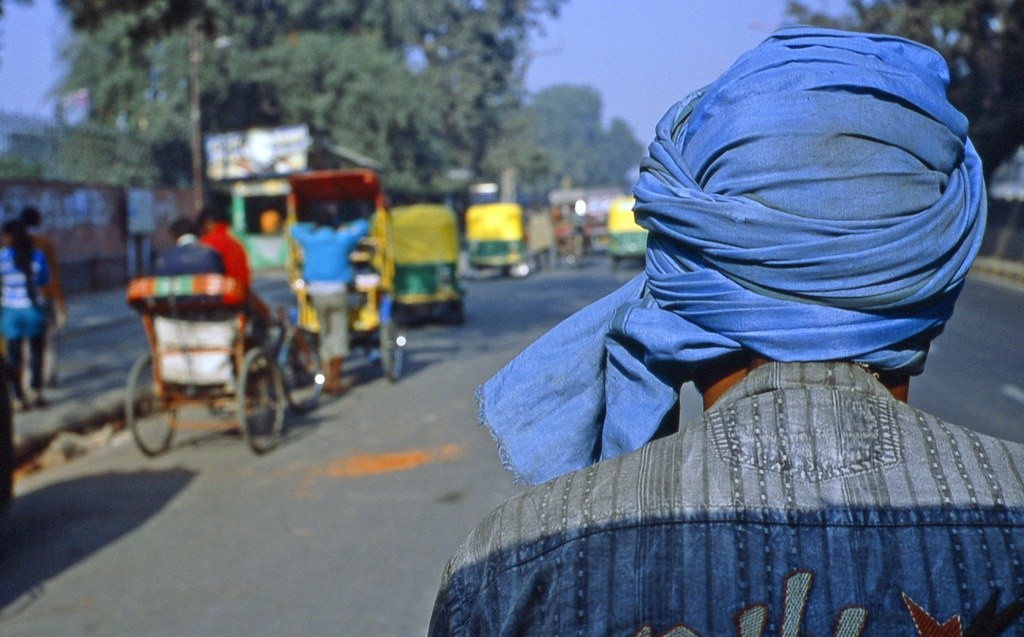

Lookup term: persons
[288,212,372,396]
[160,209,251,321]
[424,24,1024,637]
[0,204,67,506]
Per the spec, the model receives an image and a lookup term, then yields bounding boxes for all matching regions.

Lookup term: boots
[324,358,343,394]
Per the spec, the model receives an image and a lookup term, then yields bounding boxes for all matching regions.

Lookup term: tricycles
[121,272,334,460]
[229,169,406,389]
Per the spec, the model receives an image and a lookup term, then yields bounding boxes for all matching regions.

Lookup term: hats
[259,209,282,234]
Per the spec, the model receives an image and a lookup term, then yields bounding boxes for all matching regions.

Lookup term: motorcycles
[386,202,470,327]
[608,194,652,264]
[465,202,531,279]
